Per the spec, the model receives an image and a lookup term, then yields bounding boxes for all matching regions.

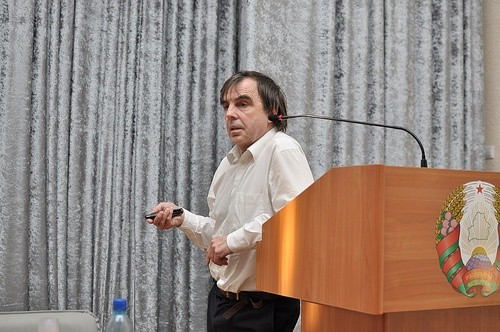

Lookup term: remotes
[145,208,183,219]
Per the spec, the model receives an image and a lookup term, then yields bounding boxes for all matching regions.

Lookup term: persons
[147,70,315,332]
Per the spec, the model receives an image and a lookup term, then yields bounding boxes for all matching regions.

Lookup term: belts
[218,287,264,320]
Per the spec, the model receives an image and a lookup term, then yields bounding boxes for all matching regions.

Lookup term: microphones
[268,113,427,167]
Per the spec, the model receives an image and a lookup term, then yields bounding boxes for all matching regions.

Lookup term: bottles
[105,298,135,331]
